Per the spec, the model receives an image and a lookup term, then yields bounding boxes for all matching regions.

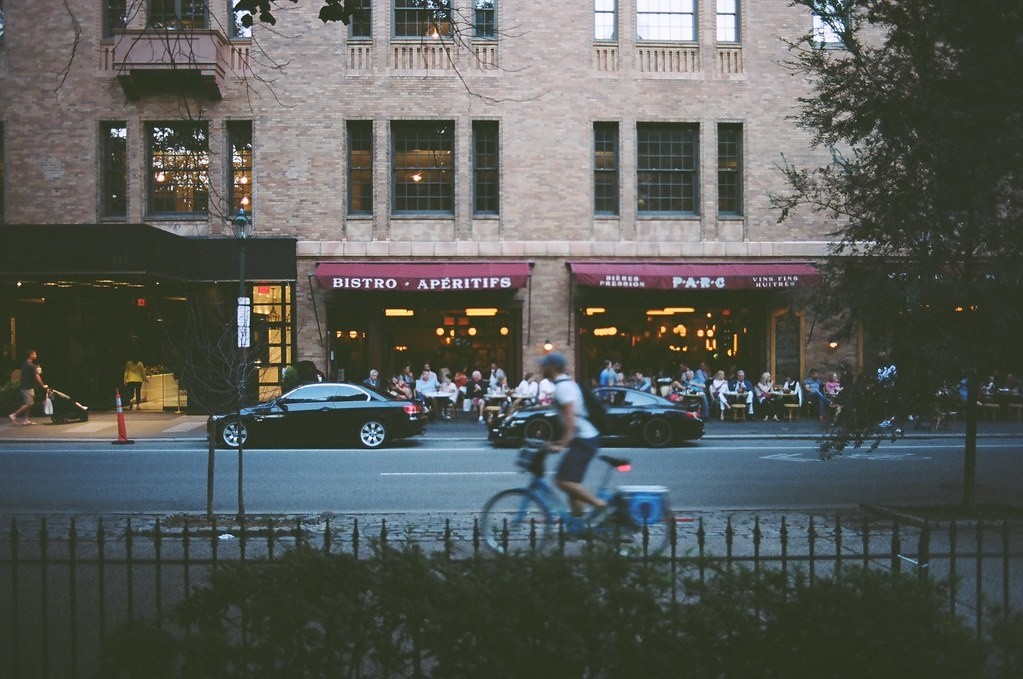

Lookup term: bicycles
[476,436,674,558]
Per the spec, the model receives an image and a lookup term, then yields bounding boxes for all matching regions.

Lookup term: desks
[487,393,507,402]
[425,392,449,423]
[680,390,705,412]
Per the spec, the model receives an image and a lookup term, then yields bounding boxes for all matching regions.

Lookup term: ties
[739,383,741,388]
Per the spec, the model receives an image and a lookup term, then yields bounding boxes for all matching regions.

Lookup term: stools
[1008,404,1023,420]
[486,406,500,423]
[732,405,747,421]
[982,404,1000,421]
[785,404,798,422]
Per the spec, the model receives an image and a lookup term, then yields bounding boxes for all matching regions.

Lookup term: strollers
[43,387,88,424]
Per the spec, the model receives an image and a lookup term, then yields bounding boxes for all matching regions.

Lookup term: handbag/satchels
[44,393,53,415]
[712,392,720,398]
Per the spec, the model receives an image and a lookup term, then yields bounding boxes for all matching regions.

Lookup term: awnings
[568,258,824,291]
[313,260,535,292]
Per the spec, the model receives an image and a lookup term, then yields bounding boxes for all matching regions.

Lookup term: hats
[537,352,566,366]
[370,369,378,376]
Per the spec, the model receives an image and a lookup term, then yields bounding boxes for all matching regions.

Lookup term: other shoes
[772,417,780,422]
[445,415,451,419]
[558,511,585,522]
[8,414,19,425]
[589,502,611,519]
[477,420,486,425]
[22,421,37,425]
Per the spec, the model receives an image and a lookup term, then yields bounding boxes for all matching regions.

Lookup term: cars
[206,383,428,450]
[490,387,705,449]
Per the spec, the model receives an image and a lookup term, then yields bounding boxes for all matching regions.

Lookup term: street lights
[231,208,255,413]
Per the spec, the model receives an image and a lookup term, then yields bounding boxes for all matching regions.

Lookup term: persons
[226,302,1023,529]
[124,350,180,409]
[8,348,49,426]
